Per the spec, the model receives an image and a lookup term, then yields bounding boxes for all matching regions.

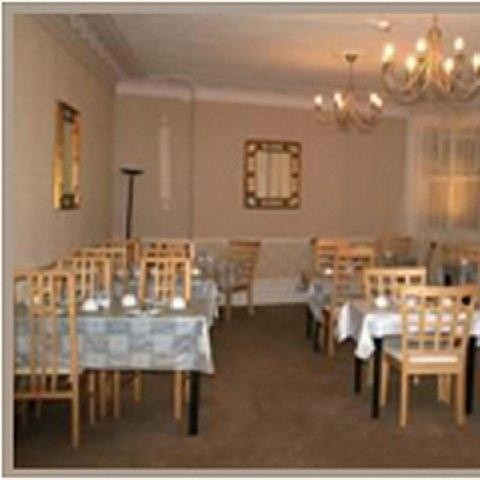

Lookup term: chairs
[12,239,261,450]
[300,238,479,432]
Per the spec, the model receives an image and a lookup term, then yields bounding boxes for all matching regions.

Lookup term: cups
[83,296,97,310]
[170,295,185,310]
[374,295,388,306]
[121,292,135,307]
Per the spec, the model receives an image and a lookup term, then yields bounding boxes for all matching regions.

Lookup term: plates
[127,308,158,316]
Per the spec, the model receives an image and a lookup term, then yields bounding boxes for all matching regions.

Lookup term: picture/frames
[50,97,81,209]
[241,137,304,210]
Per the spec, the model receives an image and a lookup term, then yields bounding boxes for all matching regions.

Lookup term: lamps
[311,15,478,135]
